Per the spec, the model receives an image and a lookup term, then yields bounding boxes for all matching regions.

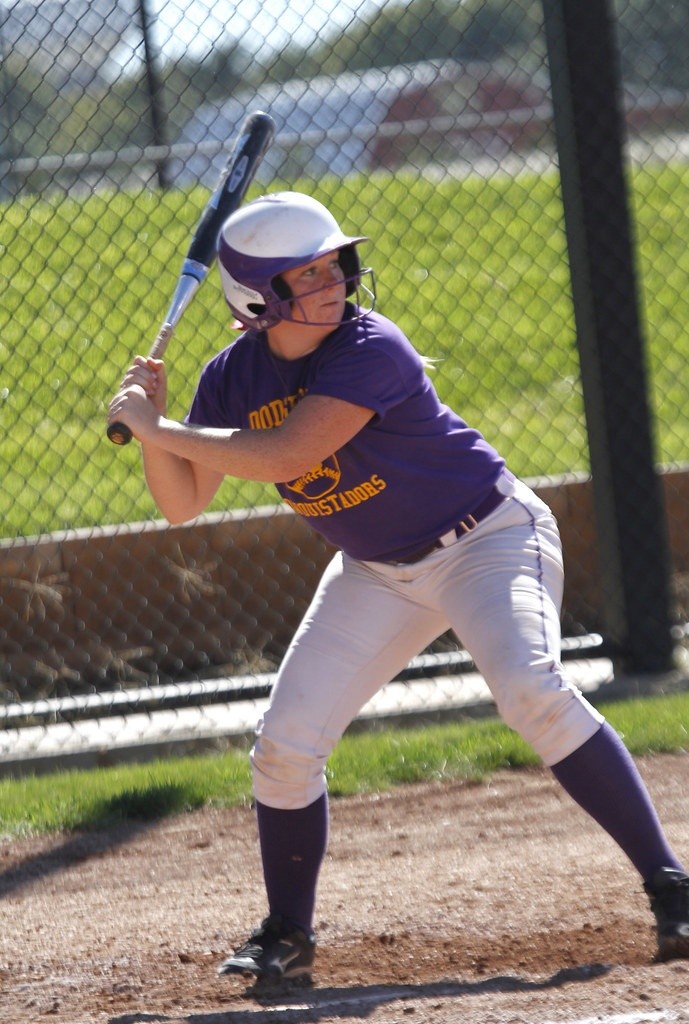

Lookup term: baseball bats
[105,110,281,445]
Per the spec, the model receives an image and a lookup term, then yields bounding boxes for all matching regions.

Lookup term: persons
[107,192,688,970]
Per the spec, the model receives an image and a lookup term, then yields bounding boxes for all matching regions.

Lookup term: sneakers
[642,868,689,958]
[220,915,316,986]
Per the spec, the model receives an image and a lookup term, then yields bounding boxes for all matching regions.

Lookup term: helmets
[217,191,368,329]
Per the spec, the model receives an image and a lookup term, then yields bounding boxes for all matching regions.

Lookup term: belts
[396,467,516,561]
